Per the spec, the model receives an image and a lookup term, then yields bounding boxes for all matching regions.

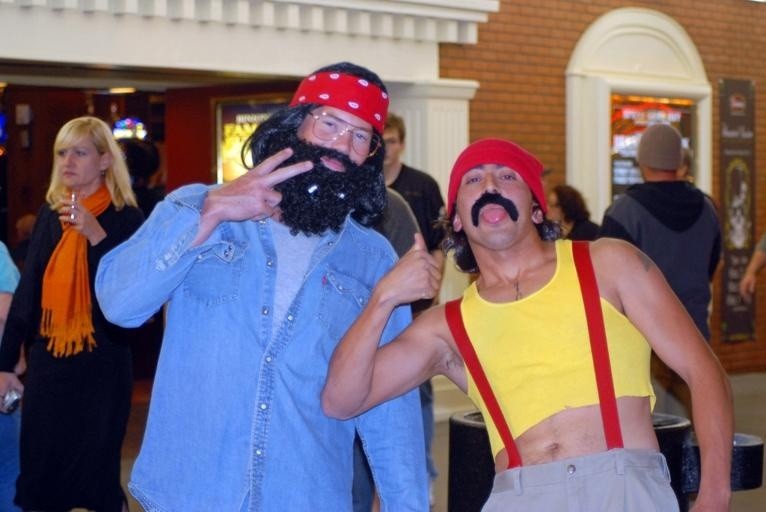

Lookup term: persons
[120,138,172,375]
[738,229,766,307]
[544,183,605,241]
[1,116,147,510]
[95,62,434,511]
[1,235,27,510]
[381,113,443,470]
[363,170,420,254]
[318,137,736,512]
[598,121,721,345]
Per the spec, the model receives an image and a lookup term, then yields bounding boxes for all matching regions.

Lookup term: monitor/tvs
[112,116,148,141]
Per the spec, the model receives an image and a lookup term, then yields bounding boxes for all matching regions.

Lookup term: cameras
[2,389,22,414]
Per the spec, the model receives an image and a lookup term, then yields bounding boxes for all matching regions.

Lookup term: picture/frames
[209,91,300,184]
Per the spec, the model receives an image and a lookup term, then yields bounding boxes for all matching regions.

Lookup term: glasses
[306,110,383,159]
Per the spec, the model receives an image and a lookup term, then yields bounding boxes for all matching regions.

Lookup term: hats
[634,123,683,171]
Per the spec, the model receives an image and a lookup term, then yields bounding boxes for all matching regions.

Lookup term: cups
[61,192,84,222]
